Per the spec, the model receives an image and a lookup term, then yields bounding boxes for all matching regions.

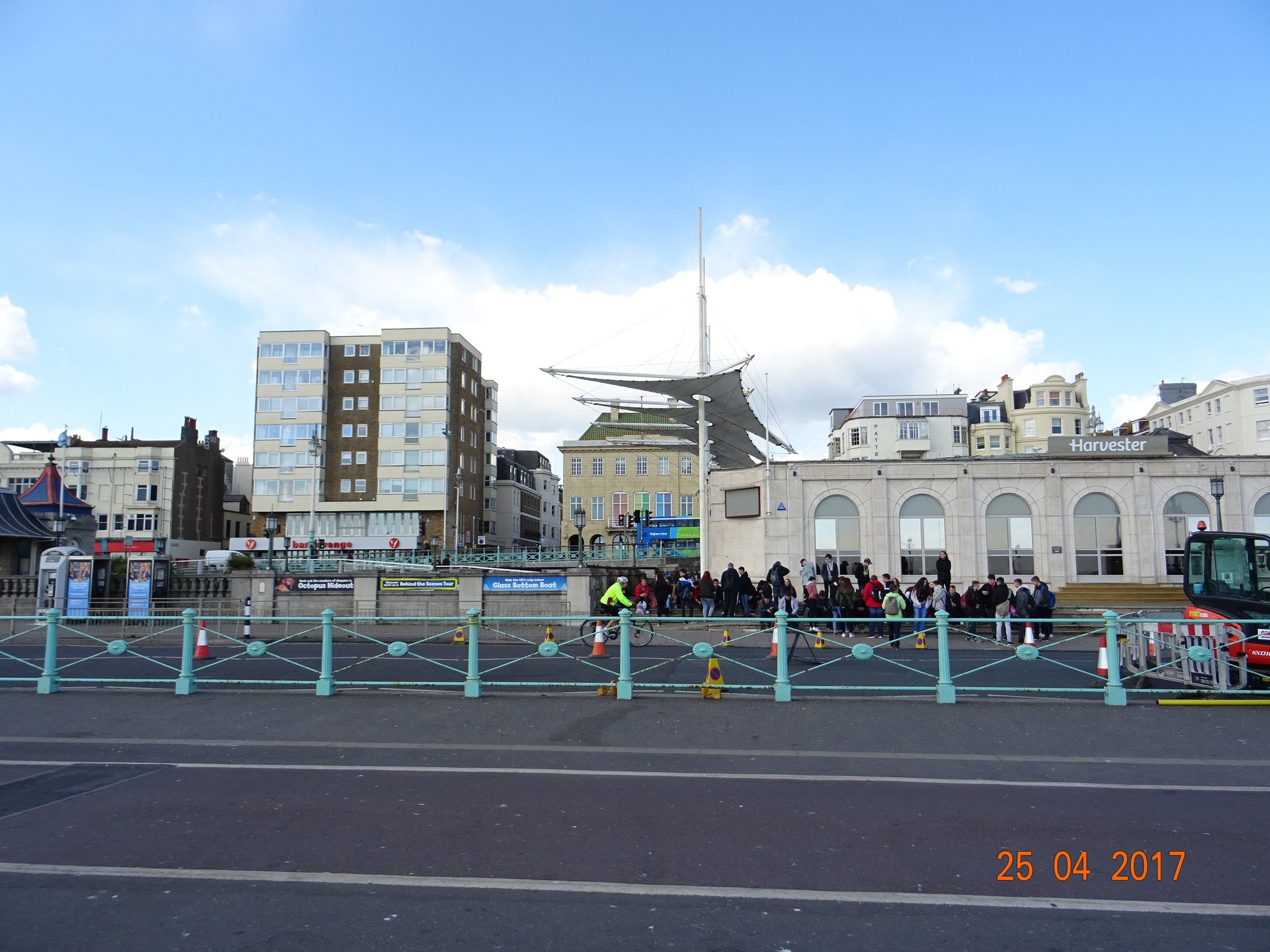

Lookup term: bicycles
[580,605,654,647]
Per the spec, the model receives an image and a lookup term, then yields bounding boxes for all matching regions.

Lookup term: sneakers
[602,628,611,638]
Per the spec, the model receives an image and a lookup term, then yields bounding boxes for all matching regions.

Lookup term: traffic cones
[1023,621,1043,659]
[705,620,826,692]
[587,620,610,659]
[1089,635,1108,689]
[915,633,928,650]
[193,620,217,660]
[451,626,465,644]
[1149,631,1156,656]
[544,626,555,643]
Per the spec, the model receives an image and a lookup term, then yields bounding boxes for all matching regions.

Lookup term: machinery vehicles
[1183,521,1270,666]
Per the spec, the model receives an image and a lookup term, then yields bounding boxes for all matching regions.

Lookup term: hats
[865,558,872,564]
[801,558,805,567]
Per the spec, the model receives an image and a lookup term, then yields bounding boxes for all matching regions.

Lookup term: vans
[204,550,249,573]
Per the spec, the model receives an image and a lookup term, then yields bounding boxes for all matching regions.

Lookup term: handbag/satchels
[635,601,647,614]
[996,600,1010,616]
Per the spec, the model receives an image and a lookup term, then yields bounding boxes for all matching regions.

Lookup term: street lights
[431,535,439,572]
[454,467,464,565]
[266,503,278,570]
[630,530,637,569]
[574,499,585,569]
[1085,405,1104,437]
[284,530,291,574]
[53,516,66,547]
[1209,465,1224,533]
[307,422,322,560]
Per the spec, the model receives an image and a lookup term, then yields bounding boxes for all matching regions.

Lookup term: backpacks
[1041,585,1055,608]
[869,582,885,601]
[885,595,900,615]
[770,568,779,586]
[682,577,692,599]
[940,589,952,612]
[840,594,853,611]
[787,614,799,629]
[1020,587,1036,611]
[802,584,813,602]
[960,590,974,609]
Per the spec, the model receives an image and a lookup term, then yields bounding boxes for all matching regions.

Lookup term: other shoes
[646,609,650,614]
[965,631,1053,646]
[810,626,927,650]
[683,622,686,624]
[657,623,662,626]
[766,624,769,628]
[688,621,697,624]
[743,615,751,617]
[758,626,765,630]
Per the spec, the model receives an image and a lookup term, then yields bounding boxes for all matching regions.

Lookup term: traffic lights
[419,521,425,536]
[319,539,324,549]
[618,510,653,529]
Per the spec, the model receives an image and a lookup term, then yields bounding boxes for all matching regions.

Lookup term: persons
[936,551,951,593]
[652,553,1056,651]
[69,562,88,579]
[423,540,431,557]
[309,545,318,560]
[600,577,637,637]
[129,562,149,581]
[434,554,450,572]
[634,578,651,614]
[346,550,355,562]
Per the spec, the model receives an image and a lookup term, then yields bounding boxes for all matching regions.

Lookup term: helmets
[617,576,628,583]
